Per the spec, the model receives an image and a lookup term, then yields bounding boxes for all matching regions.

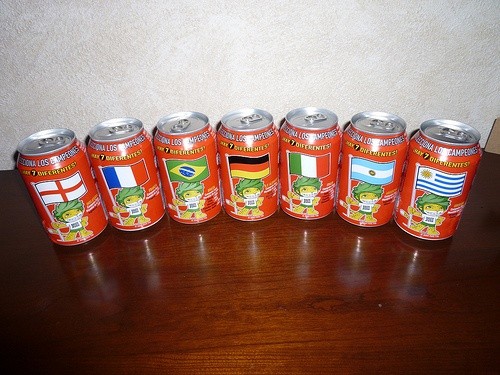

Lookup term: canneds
[392,118,483,240]
[335,111,409,228]
[154,111,223,224]
[86,117,166,231]
[280,107,343,220]
[216,108,280,221]
[16,128,109,246]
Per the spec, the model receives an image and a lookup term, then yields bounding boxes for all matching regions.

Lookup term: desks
[0,148,500,375]
